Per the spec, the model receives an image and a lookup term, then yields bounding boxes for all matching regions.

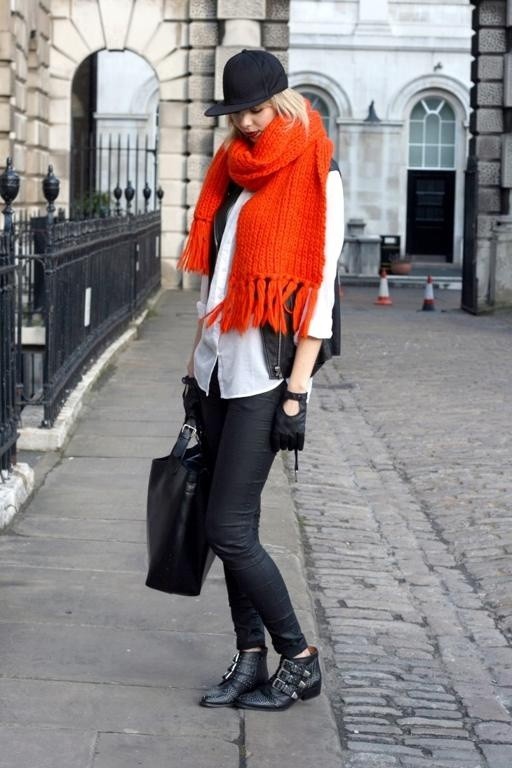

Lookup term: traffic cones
[374,267,394,306]
[417,273,437,311]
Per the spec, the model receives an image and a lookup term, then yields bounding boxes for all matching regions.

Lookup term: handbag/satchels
[144,411,216,597]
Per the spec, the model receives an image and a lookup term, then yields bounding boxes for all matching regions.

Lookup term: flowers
[388,253,417,263]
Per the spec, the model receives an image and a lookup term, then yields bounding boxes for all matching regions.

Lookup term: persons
[180,50,346,710]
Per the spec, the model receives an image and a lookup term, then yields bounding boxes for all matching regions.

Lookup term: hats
[204,49,288,117]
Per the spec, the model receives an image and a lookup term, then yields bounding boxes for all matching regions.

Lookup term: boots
[199,647,269,707]
[234,646,322,712]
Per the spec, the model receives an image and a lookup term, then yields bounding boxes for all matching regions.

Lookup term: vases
[391,261,412,274]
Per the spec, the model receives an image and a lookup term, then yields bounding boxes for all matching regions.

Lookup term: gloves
[182,376,203,417]
[270,389,307,452]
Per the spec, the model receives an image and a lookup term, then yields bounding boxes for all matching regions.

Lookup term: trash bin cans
[380,235,400,274]
